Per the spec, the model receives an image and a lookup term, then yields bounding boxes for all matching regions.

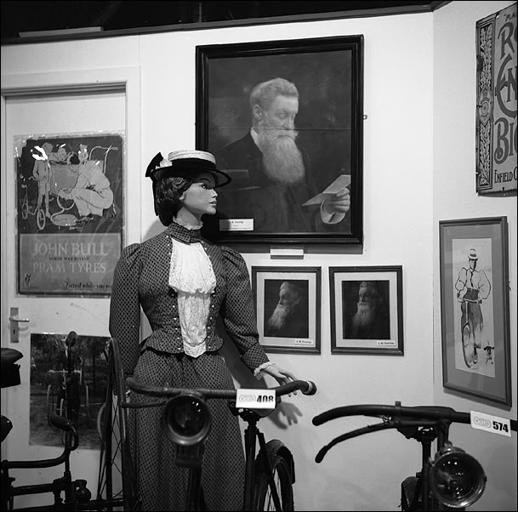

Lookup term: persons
[343,282,389,339]
[454,249,492,346]
[47,339,86,419]
[212,77,351,236]
[264,280,308,337]
[109,148,297,510]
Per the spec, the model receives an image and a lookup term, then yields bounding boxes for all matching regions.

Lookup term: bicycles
[311,398,517,511]
[18,173,46,232]
[457,293,487,368]
[117,375,317,511]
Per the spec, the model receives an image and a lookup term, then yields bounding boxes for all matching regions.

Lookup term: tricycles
[45,370,93,431]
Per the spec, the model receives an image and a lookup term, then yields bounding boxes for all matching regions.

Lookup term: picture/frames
[250,265,321,354]
[329,266,404,355]
[193,33,365,246]
[439,216,512,407]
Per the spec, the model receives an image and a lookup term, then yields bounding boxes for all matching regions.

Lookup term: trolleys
[48,145,118,211]
